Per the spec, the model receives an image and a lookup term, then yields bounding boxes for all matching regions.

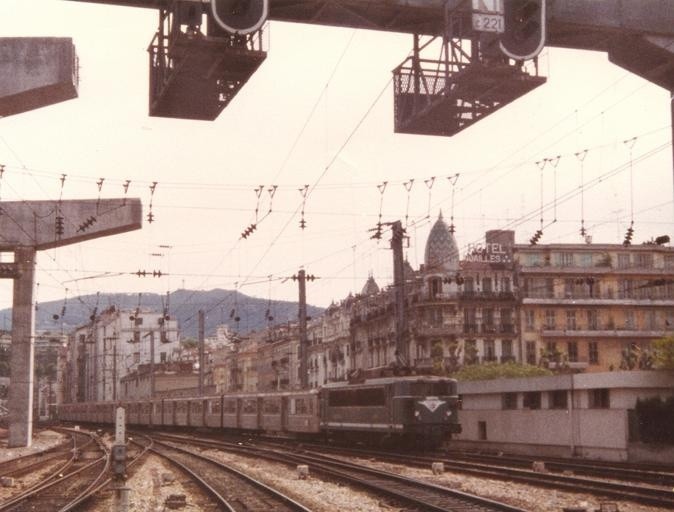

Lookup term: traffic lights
[228,1,253,16]
[508,0,539,44]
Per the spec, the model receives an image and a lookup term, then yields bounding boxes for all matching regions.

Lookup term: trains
[53,372,465,452]
